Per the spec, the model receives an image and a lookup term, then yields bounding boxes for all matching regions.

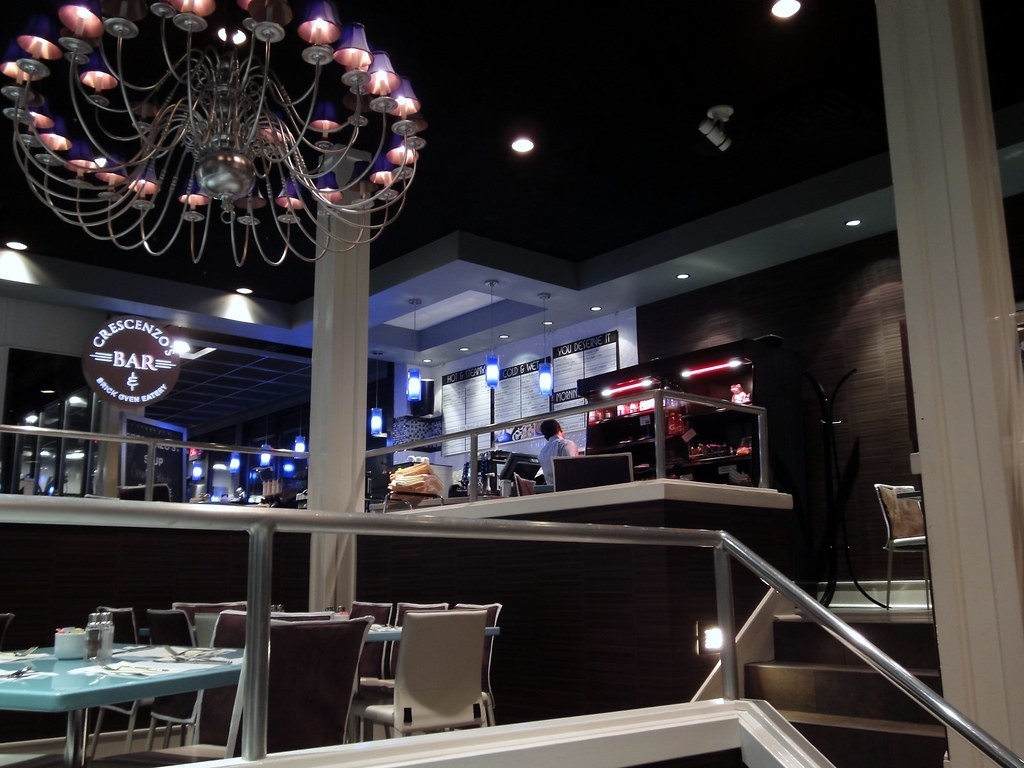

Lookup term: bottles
[263,478,282,494]
[588,386,692,423]
[83,612,114,664]
[467,477,483,495]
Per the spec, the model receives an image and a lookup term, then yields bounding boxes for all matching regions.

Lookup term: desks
[0,644,251,768]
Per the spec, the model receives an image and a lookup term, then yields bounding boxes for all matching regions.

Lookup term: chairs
[79,599,503,766]
[871,482,931,609]
[0,613,15,651]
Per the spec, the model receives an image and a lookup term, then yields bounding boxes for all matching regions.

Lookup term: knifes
[112,645,150,655]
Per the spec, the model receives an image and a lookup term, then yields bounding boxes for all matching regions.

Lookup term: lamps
[537,292,554,396]
[405,298,421,402]
[699,106,733,151]
[370,350,383,435]
[0,0,424,267]
[236,271,254,295]
[485,280,499,389]
[191,404,305,481]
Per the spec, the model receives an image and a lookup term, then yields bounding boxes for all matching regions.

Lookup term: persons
[538,420,578,484]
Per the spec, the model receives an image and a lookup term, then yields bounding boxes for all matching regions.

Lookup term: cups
[501,479,511,497]
[55,632,85,659]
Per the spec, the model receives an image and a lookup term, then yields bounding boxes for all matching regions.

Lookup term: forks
[89,656,168,671]
[14,646,38,656]
[164,644,216,655]
[0,666,32,678]
